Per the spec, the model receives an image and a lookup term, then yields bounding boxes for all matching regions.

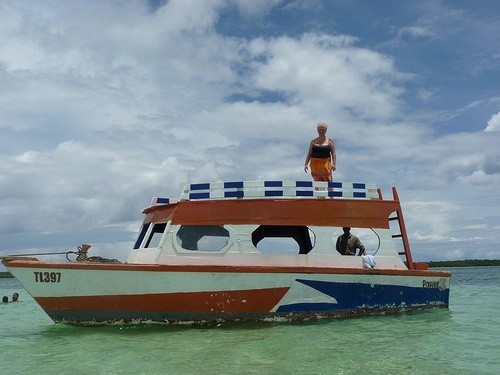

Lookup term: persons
[2,296,8,303]
[304,123,336,199]
[336,227,365,256]
[12,293,19,301]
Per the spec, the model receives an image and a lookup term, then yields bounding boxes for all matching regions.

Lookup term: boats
[1,179,453,327]
